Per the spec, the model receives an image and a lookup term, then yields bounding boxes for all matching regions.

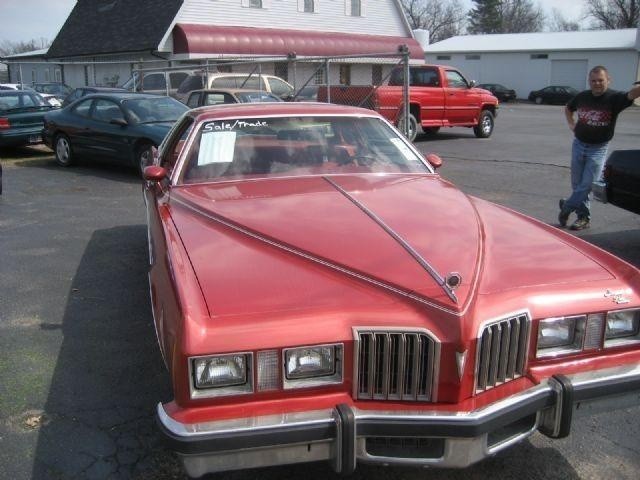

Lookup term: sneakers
[558,199,571,225]
[572,215,591,230]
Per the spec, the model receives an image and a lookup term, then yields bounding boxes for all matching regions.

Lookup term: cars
[41,92,191,179]
[529,86,582,105]
[142,101,640,479]
[0,82,129,154]
[475,84,516,102]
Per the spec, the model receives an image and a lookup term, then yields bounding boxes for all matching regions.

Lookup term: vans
[175,73,296,108]
[117,70,218,97]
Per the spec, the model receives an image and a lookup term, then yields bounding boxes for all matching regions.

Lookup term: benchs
[80,107,120,122]
[170,138,357,180]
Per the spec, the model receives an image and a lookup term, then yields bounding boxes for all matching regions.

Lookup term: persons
[557,65,640,232]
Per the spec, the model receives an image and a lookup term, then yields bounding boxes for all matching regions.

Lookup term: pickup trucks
[318,64,498,144]
